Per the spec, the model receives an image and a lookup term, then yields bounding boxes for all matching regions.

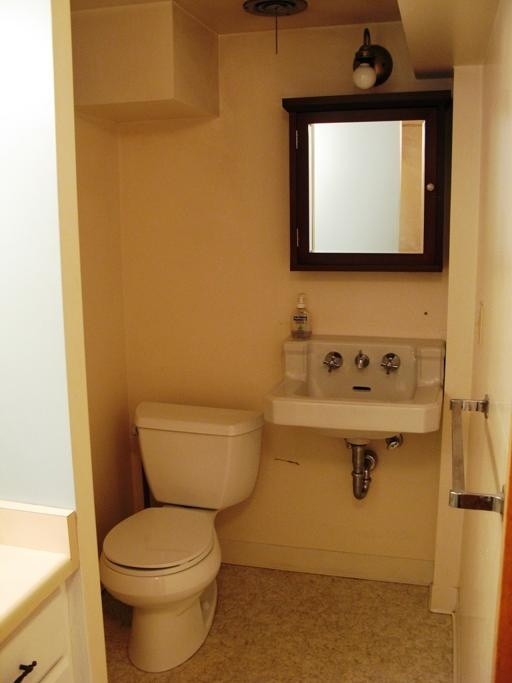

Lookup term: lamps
[351,28,393,90]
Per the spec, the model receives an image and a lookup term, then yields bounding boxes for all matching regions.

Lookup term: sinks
[264,378,442,445]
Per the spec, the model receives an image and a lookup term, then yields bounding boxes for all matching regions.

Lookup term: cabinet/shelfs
[1,584,80,683]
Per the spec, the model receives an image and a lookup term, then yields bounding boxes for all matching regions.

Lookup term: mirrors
[290,108,444,272]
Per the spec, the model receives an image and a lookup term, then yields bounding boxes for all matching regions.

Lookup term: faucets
[355,349,371,370]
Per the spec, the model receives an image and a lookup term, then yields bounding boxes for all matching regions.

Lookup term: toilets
[100,399,263,673]
